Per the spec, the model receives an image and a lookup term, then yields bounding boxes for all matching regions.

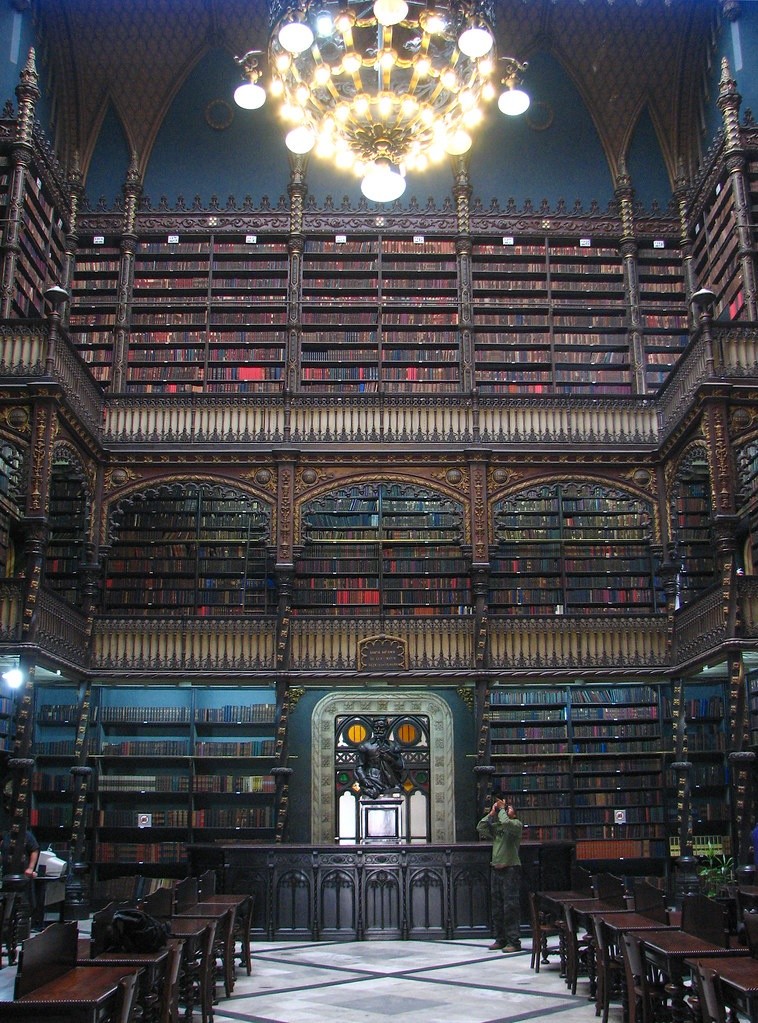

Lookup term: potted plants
[698,839,739,934]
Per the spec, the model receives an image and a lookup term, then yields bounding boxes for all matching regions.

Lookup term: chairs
[526,891,580,973]
[621,932,699,1023]
[560,901,618,995]
[696,961,741,1023]
[113,894,256,1023]
[592,914,657,1023]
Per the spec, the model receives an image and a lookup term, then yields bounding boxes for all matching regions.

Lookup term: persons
[476,798,522,953]
[26,831,39,919]
[353,718,403,799]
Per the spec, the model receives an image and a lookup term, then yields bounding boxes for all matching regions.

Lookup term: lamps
[233,0,531,203]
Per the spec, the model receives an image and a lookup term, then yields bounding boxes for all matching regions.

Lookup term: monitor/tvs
[34,851,68,877]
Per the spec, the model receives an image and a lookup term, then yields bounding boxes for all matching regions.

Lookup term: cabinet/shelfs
[0,150,758,916]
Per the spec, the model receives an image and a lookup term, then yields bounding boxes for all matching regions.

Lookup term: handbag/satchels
[111,908,171,953]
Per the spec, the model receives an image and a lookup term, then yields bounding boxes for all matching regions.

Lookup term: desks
[141,886,218,1023]
[89,897,186,1023]
[173,877,234,993]
[199,869,250,981]
[538,891,758,1023]
[0,920,146,1023]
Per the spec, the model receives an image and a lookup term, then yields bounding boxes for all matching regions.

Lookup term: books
[748,679,758,692]
[36,703,280,864]
[688,724,724,751]
[481,687,672,857]
[88,877,181,899]
[0,161,758,393]
[752,696,758,710]
[0,499,711,615]
[0,680,14,751]
[686,697,724,717]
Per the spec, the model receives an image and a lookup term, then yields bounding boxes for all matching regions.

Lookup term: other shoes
[489,942,507,950]
[502,943,521,952]
